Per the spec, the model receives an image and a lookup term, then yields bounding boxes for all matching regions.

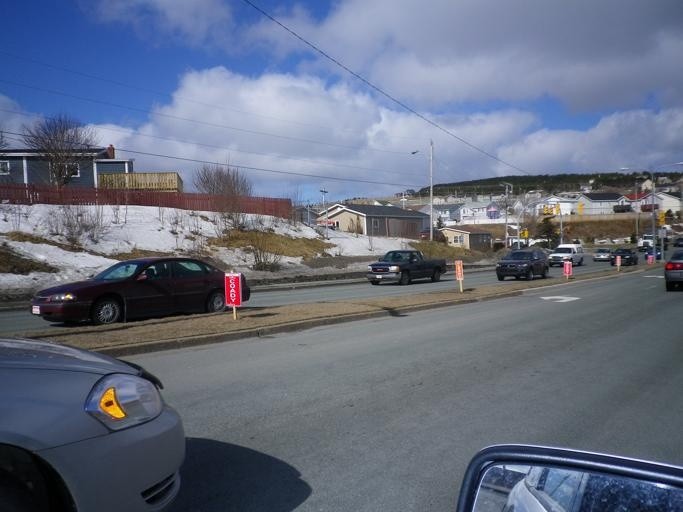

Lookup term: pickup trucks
[366,250,446,285]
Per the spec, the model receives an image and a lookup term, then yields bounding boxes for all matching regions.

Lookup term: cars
[501,466,683,512]
[496,244,584,280]
[0,338,186,512]
[593,234,683,289]
[30,257,250,326]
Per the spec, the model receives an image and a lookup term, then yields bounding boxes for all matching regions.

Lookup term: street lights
[620,162,683,263]
[411,139,433,241]
